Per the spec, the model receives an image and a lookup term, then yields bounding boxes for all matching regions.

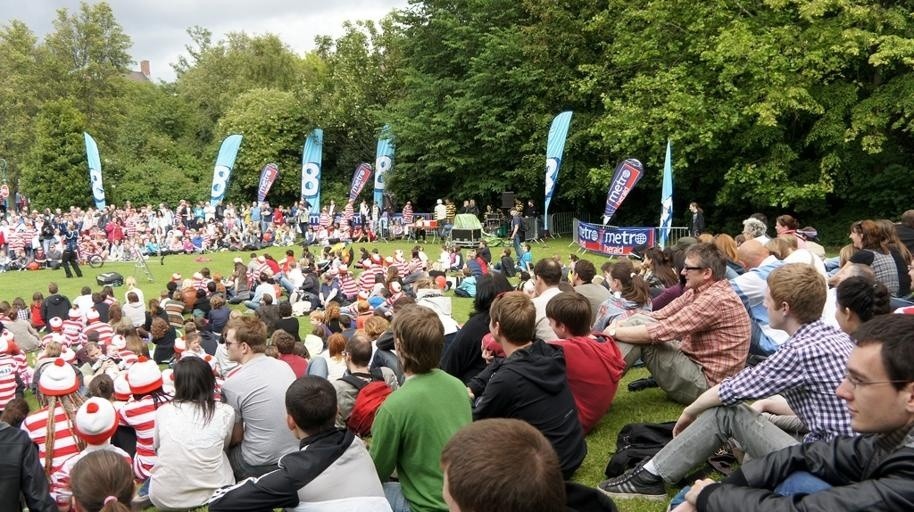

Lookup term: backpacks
[602,421,712,480]
[345,379,395,436]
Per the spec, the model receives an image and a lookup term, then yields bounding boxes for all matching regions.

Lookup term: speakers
[502,192,514,208]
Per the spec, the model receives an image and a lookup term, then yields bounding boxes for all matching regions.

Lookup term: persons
[687,204,705,237]
[0,178,537,277]
[0,212,914,511]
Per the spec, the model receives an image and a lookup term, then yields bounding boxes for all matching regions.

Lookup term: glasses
[682,261,704,271]
[224,339,241,348]
[840,366,910,391]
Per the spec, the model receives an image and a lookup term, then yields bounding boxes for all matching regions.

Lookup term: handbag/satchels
[527,262,534,270]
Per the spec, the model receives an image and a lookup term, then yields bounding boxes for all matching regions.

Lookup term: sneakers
[598,462,670,501]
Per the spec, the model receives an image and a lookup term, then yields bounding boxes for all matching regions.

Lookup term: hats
[385,256,394,264]
[111,334,126,350]
[0,341,11,354]
[363,258,372,267]
[1,327,14,341]
[395,249,403,256]
[389,281,402,294]
[396,256,402,263]
[338,264,347,273]
[37,359,79,397]
[257,255,266,262]
[73,394,119,445]
[172,273,181,280]
[359,290,368,299]
[67,305,81,319]
[372,254,380,261]
[124,355,163,395]
[192,271,203,280]
[47,316,63,330]
[85,308,100,321]
